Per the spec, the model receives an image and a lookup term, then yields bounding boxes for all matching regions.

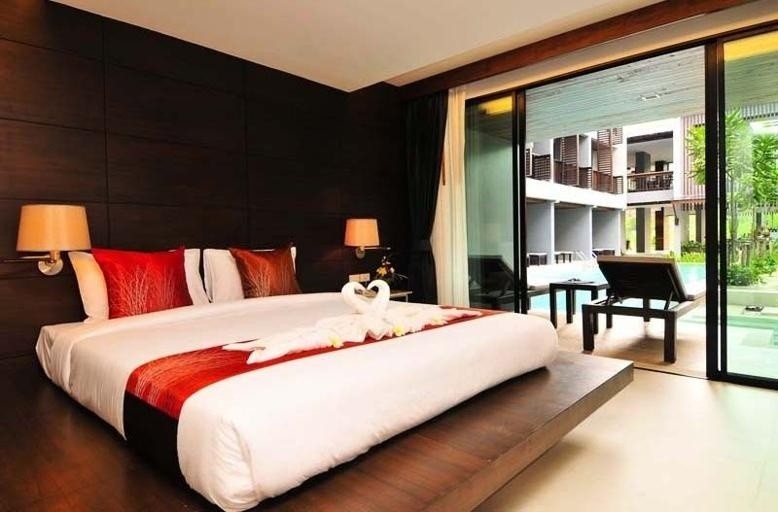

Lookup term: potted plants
[371,255,409,291]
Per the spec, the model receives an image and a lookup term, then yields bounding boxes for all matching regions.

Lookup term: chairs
[468,248,706,364]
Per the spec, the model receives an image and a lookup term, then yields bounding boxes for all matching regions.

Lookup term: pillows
[67,238,304,324]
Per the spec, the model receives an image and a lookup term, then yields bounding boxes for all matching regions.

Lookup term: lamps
[344,218,392,259]
[0,204,92,276]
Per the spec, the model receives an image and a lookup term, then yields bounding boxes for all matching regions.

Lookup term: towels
[366,279,483,337]
[221,281,394,365]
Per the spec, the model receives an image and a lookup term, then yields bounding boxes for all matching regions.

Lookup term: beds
[35,292,559,512]
